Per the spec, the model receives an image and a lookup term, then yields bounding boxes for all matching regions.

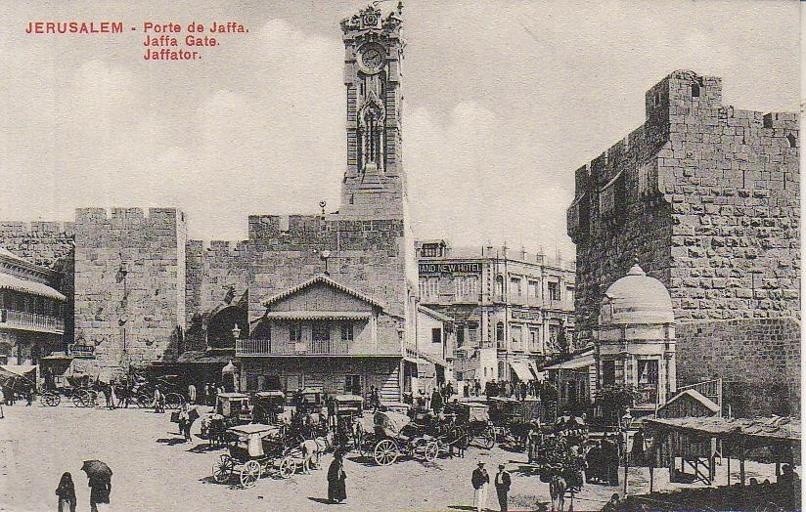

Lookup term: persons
[87,478,112,512]
[326,448,348,504]
[154,382,226,443]
[0,389,4,419]
[368,384,375,408]
[430,379,454,416]
[372,387,382,414]
[108,379,117,410]
[471,460,490,512]
[494,463,512,511]
[586,426,645,486]
[55,471,77,512]
[463,378,545,401]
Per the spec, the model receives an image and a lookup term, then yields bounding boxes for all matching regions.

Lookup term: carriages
[0,372,225,412]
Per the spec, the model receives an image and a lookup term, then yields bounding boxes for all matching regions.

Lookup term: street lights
[621,409,633,494]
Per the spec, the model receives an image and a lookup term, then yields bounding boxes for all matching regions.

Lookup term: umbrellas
[80,459,114,478]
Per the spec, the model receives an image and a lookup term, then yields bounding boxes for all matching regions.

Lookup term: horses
[301,432,332,475]
[433,417,470,460]
